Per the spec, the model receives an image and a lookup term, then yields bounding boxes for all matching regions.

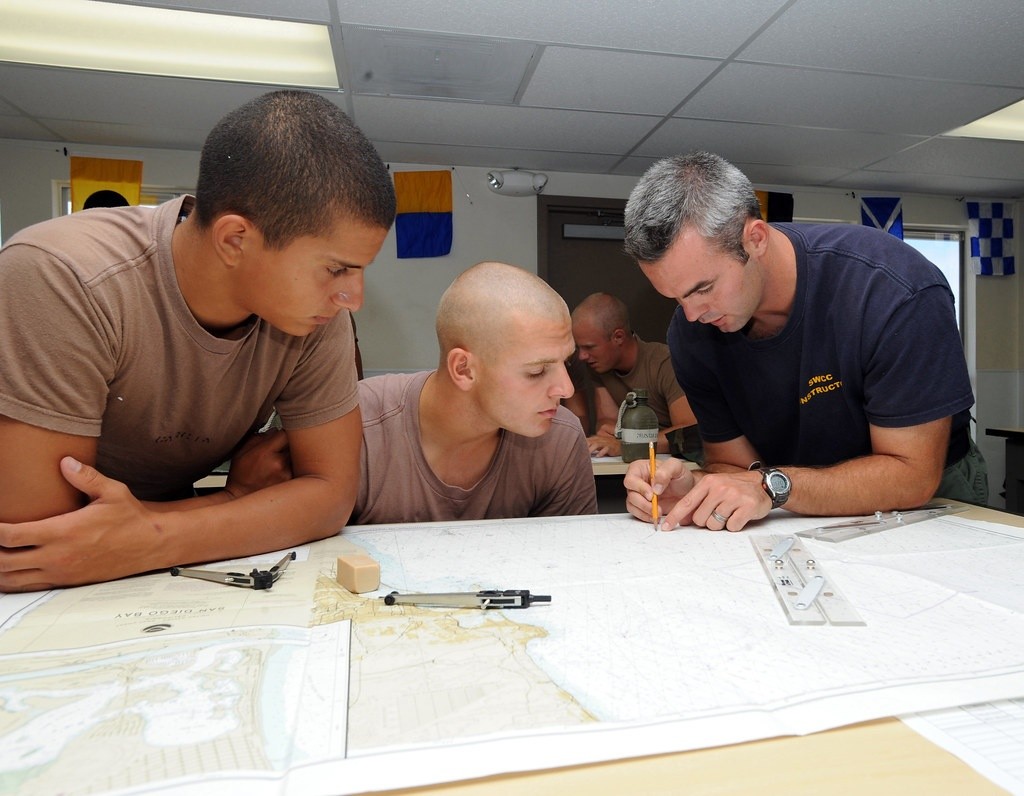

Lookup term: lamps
[485,169,549,196]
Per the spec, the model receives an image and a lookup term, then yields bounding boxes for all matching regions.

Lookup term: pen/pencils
[649,440,659,532]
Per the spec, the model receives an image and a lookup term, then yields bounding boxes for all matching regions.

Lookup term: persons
[0,89,400,594]
[624,152,990,532]
[346,261,599,526]
[561,292,698,459]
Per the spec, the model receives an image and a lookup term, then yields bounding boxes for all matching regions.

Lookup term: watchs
[748,460,792,510]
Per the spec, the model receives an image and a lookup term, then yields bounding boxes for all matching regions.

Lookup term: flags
[753,189,795,224]
[391,170,454,259]
[67,153,142,213]
[859,194,904,240]
[966,200,1016,277]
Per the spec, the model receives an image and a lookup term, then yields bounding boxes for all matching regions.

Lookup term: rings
[711,510,728,522]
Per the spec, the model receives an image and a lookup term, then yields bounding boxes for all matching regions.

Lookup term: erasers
[334,551,381,595]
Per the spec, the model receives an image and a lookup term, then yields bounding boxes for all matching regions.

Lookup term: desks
[0,428,1024,795]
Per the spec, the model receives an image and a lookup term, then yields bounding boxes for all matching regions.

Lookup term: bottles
[614,388,659,462]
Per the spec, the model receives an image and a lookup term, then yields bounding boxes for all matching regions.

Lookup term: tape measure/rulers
[795,502,972,544]
[748,529,868,629]
